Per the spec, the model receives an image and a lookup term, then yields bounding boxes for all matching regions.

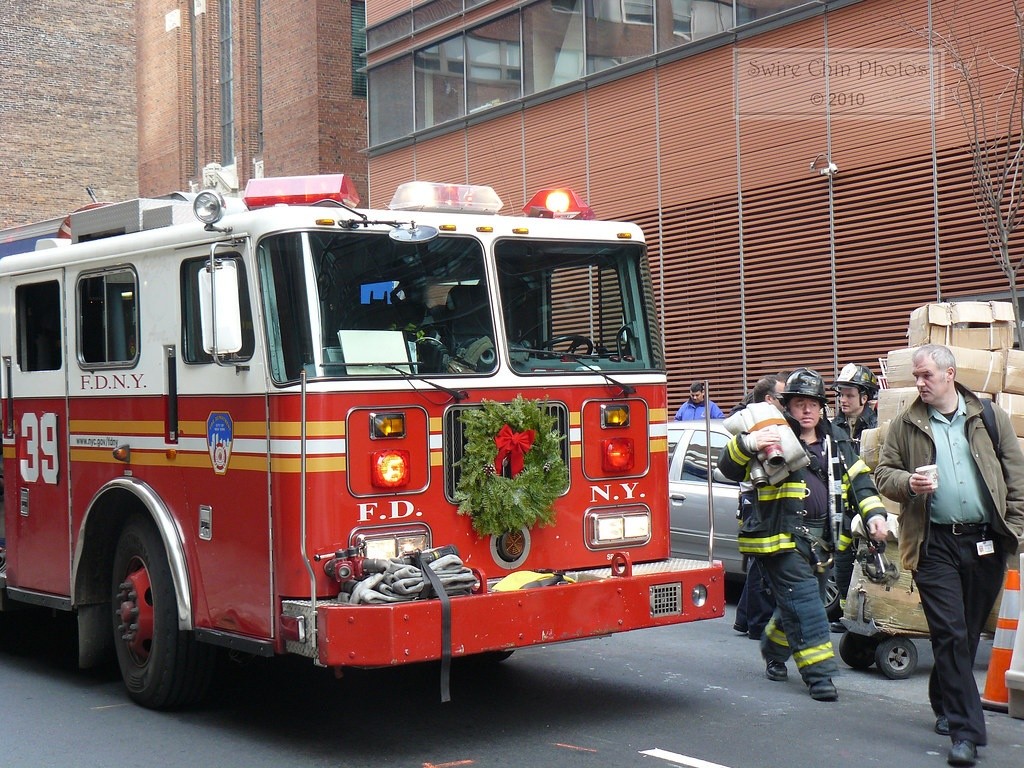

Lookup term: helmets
[781,368,830,405]
[832,363,880,400]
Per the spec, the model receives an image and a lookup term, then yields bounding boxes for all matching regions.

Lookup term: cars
[668,421,845,617]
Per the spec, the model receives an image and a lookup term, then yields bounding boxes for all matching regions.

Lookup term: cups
[915,464,938,489]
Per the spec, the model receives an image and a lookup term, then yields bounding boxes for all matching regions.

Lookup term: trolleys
[837,588,999,681]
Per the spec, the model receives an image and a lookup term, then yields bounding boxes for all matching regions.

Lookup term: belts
[929,520,994,536]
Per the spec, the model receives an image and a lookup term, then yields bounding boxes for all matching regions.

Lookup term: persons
[821,358,879,635]
[672,381,725,421]
[727,372,790,642]
[871,343,1023,764]
[711,367,889,705]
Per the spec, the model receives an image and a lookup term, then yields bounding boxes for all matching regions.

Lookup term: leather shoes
[733,622,763,640]
[830,622,846,634]
[810,678,839,700]
[765,654,788,680]
[936,713,954,736]
[947,739,979,765]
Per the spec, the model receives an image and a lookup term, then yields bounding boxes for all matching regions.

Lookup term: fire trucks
[1,174,724,711]
